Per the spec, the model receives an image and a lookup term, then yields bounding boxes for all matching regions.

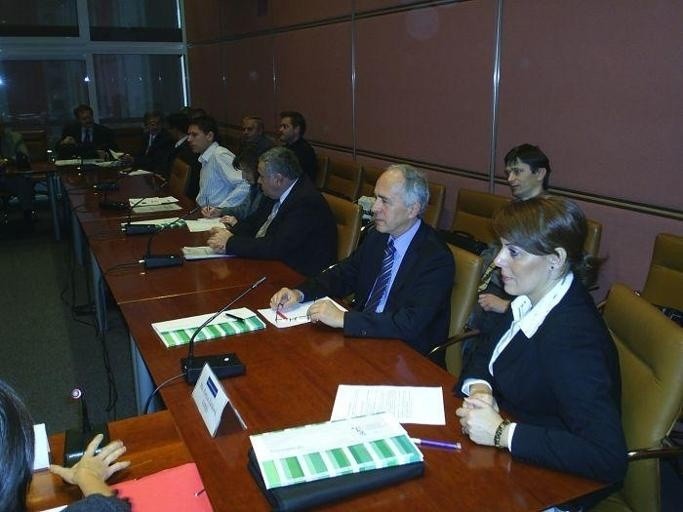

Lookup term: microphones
[125,181,169,234]
[179,276,267,384]
[92,151,133,191]
[102,166,137,209]
[145,206,200,268]
[65,387,109,468]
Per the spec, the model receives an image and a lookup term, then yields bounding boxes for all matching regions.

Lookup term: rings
[461,425,466,434]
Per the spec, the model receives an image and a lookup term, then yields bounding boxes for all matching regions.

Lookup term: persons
[205,147,337,278]
[477,143,554,293]
[124,105,316,234]
[452,198,628,511]
[0,378,132,511]
[0,118,41,228]
[269,162,454,373]
[54,104,118,154]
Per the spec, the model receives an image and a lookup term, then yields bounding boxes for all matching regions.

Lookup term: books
[119,216,185,230]
[149,306,266,348]
[245,409,425,512]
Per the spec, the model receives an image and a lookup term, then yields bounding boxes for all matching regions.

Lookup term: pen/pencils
[411,437,462,450]
[206,196,210,213]
[313,298,318,322]
[226,313,245,324]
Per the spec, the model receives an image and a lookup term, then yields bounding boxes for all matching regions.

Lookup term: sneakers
[0,208,9,224]
[24,208,40,224]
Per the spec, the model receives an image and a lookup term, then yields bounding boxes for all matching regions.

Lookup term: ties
[361,239,397,314]
[82,129,91,154]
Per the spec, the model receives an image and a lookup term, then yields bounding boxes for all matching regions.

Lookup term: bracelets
[3,158,8,165]
[493,418,510,449]
